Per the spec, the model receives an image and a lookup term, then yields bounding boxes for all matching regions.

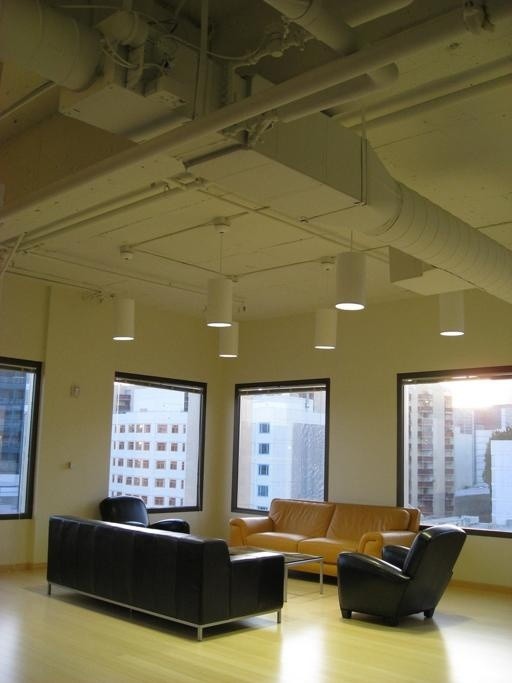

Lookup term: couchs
[47,514,285,642]
[227,497,422,577]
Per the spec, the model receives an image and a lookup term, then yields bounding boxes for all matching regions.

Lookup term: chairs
[100,495,191,534]
[336,523,466,627]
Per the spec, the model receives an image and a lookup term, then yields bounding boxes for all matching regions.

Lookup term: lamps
[114,297,136,341]
[314,306,338,349]
[335,251,366,311]
[219,323,240,357]
[205,278,235,328]
[438,291,465,337]
